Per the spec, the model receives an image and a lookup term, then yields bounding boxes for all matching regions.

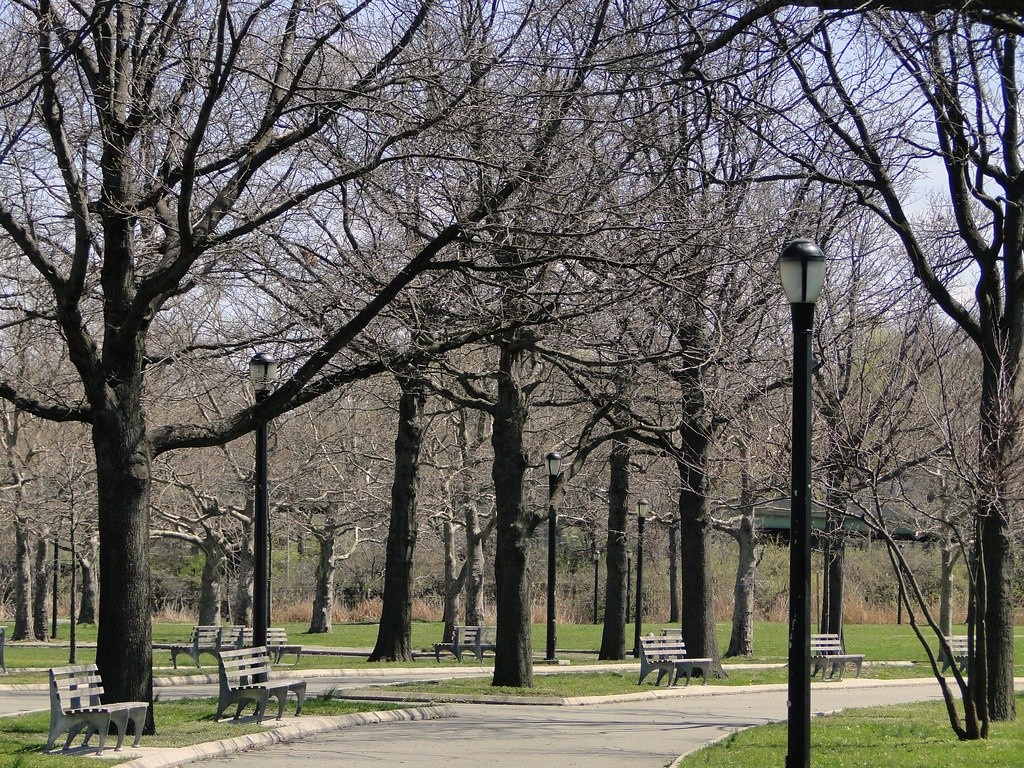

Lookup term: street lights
[778,237,826,768]
[543,452,563,662]
[593,551,601,625]
[626,549,633,625]
[248,352,276,683]
[634,496,649,659]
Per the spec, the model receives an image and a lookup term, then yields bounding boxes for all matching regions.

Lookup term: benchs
[654,629,684,659]
[431,625,497,662]
[639,634,714,688]
[44,662,150,754]
[151,625,246,669]
[940,635,986,672]
[217,646,308,724]
[810,633,866,680]
[231,628,302,664]
